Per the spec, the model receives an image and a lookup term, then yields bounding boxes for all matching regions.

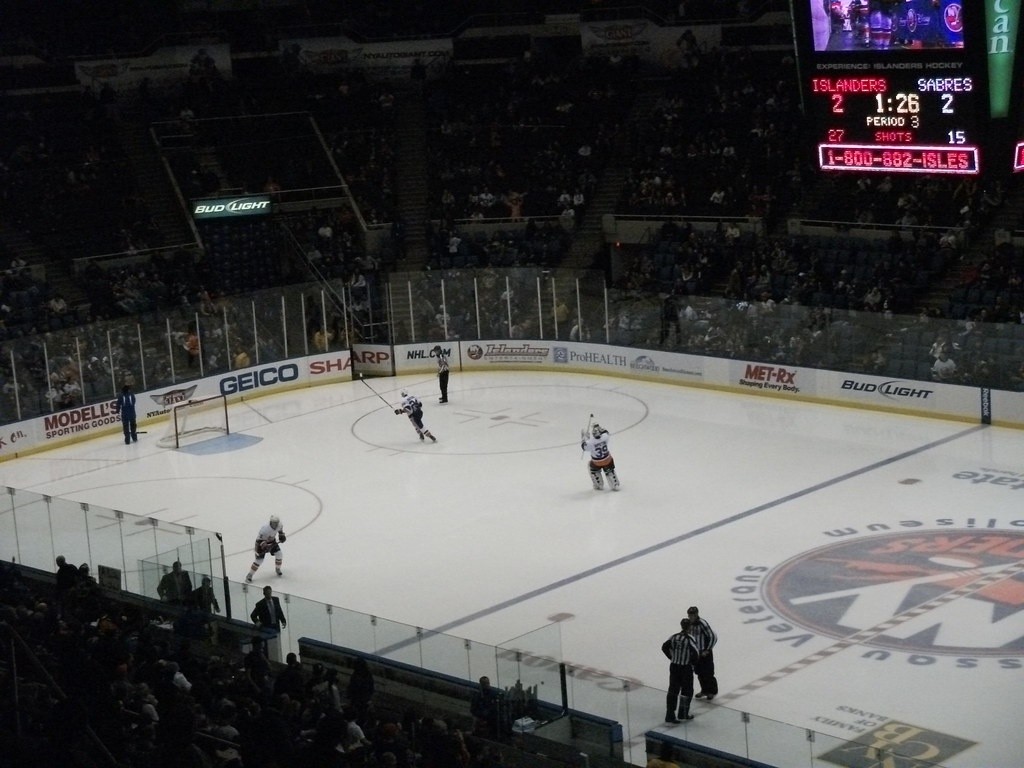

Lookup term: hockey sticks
[581,413,594,461]
[359,372,395,410]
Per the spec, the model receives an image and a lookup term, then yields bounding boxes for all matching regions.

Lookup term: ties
[268,601,276,624]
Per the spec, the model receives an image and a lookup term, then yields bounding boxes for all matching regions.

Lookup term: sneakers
[419,433,424,440]
[429,435,438,443]
[665,719,681,727]
[707,694,716,703]
[276,567,282,577]
[695,692,707,700]
[245,573,252,584]
[678,713,694,722]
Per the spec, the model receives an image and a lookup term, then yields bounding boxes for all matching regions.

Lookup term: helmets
[680,618,690,627]
[687,607,698,613]
[270,516,280,522]
[433,346,441,351]
[591,422,601,437]
[400,388,408,396]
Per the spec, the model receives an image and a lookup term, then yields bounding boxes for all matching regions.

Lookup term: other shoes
[439,398,442,400]
[439,401,448,403]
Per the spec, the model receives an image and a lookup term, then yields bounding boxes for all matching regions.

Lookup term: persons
[116,386,137,444]
[73,563,96,583]
[0,0,1024,429]
[157,562,192,600]
[191,578,220,614]
[245,515,286,583]
[56,556,78,576]
[0,565,679,768]
[662,618,699,723]
[434,346,449,403]
[581,423,621,491]
[250,586,286,631]
[687,607,718,699]
[395,389,436,441]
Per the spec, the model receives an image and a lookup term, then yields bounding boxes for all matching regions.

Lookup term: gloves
[279,533,286,543]
[395,409,399,415]
[263,545,271,553]
